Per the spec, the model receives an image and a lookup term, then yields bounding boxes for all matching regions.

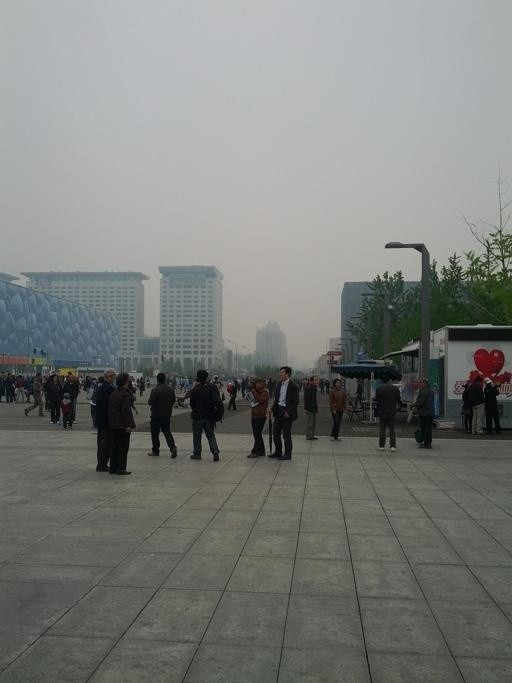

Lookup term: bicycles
[175,386,182,394]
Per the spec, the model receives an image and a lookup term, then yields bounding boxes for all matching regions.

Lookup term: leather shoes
[247,452,292,461]
[95,467,131,474]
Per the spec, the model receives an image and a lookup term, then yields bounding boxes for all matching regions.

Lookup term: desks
[344,408,362,423]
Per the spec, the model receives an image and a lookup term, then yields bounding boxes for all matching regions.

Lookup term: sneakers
[148,451,159,456]
[190,454,201,460]
[169,446,177,459]
[212,450,219,462]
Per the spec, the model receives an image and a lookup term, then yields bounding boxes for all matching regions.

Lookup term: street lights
[335,292,390,368]
[383,239,431,407]
[223,338,288,376]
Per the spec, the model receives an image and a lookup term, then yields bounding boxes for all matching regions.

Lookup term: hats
[104,368,119,375]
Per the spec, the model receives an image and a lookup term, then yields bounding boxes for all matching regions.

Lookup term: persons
[227,366,320,461]
[89,367,225,475]
[459,376,501,436]
[318,375,436,453]
[2,371,99,430]
